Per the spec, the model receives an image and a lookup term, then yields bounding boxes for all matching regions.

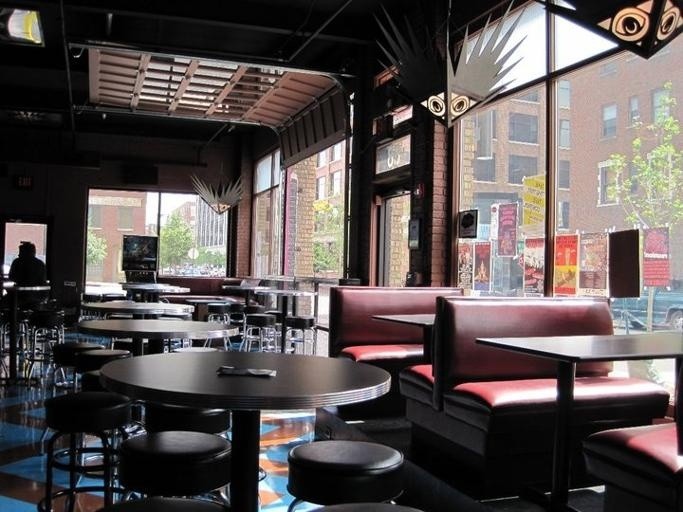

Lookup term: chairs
[93,496,235,512]
[0,278,318,402]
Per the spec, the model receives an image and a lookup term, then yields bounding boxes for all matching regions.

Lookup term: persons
[0,240,47,325]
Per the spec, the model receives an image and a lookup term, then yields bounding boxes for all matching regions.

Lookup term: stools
[131,400,267,502]
[284,440,409,512]
[80,369,143,477]
[119,428,234,512]
[38,391,142,511]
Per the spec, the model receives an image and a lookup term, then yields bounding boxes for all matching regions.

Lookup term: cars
[610,285,683,330]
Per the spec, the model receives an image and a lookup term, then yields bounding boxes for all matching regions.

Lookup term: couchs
[582,422,681,512]
[331,287,473,419]
[397,297,672,464]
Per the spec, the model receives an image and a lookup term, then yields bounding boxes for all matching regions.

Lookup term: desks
[99,349,392,510]
[475,330,682,511]
[371,311,436,364]
